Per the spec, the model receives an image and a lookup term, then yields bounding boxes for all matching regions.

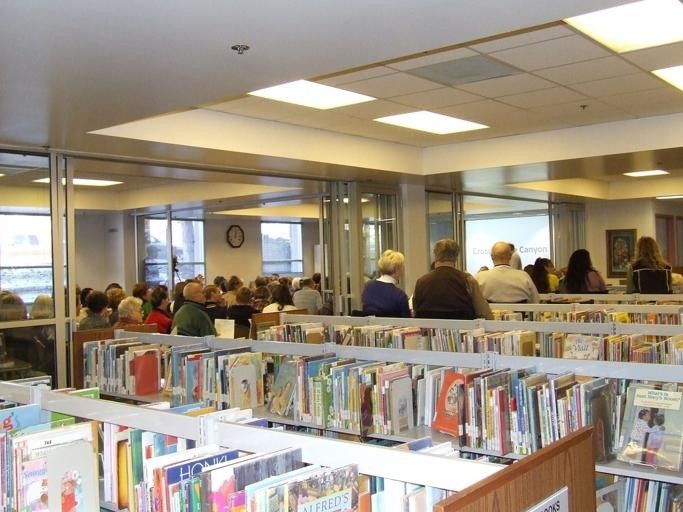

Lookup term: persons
[1,236,672,379]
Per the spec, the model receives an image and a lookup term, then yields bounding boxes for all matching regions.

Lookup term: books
[0,295,683,512]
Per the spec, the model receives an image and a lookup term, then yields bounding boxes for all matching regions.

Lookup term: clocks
[227,225,244,248]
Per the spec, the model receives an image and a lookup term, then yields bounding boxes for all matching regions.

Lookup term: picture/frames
[606,229,637,278]
